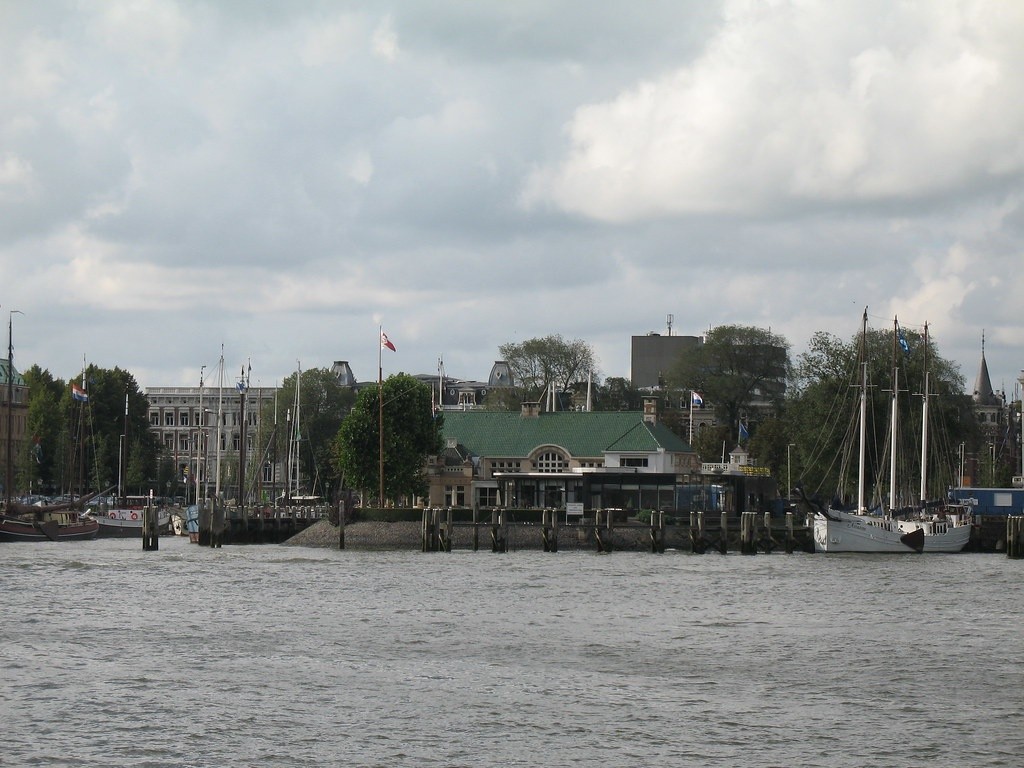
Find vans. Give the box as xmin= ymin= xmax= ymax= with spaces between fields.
xmin=1011 ymin=476 xmax=1022 ymax=488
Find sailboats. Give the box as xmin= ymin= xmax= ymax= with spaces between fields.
xmin=0 ymin=310 xmax=332 ymax=543
xmin=791 ymin=306 xmax=972 ymax=552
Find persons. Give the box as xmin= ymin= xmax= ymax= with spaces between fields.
xmin=512 ymin=495 xmax=516 ymax=507
xmin=265 ymin=505 xmax=271 ymax=519
xmin=947 ymin=483 xmax=955 ymax=504
xmin=47 ymin=513 xmax=53 ymax=522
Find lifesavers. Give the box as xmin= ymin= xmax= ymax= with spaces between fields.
xmin=131 ymin=513 xmax=138 ymax=519
xmin=109 ymin=513 xmax=116 ymax=519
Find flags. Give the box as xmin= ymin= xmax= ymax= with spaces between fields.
xmin=32 ymin=433 xmax=40 ymax=444
xmin=739 ymin=422 xmax=749 ymax=439
xmin=72 ymin=382 xmax=88 ymax=401
xmin=30 ymin=442 xmax=43 ymax=464
xmin=381 ymin=331 xmax=396 ymax=353
xmin=898 ymin=328 xmax=909 ymax=352
xmin=692 ymin=390 xmax=705 ymax=407
xmin=183 ymin=466 xmax=189 ymax=484
